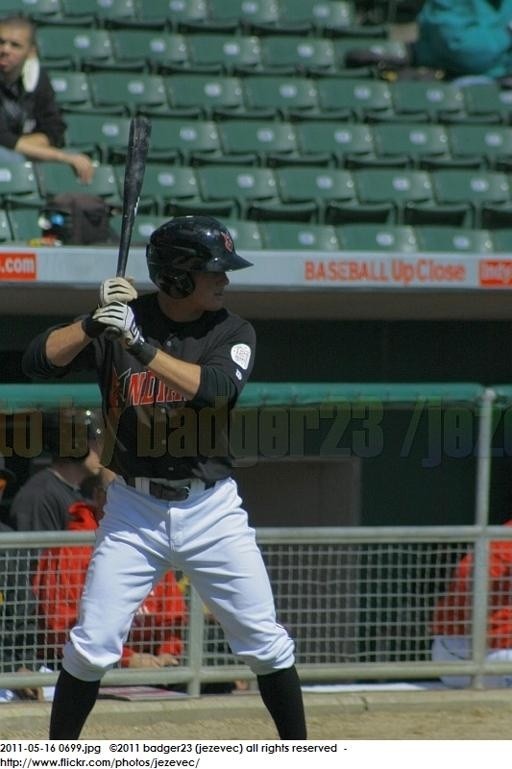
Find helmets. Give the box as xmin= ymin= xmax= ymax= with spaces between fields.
xmin=46 ymin=409 xmax=110 ymax=465
xmin=147 ymin=215 xmax=254 ymax=275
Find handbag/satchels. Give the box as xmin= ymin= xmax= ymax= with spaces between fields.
xmin=43 ymin=191 xmax=115 ymax=248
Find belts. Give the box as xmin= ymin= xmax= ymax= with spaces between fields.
xmin=121 ymin=472 xmax=217 ymax=502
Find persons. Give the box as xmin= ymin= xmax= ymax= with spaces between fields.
xmin=0 ymin=15 xmax=95 ymax=187
xmin=7 ymin=409 xmax=108 ymax=531
xmin=31 ymin=476 xmax=243 ymax=695
xmin=429 ymin=520 xmax=512 ymax=689
xmin=0 ymin=456 xmax=59 ymax=700
xmin=21 ymin=213 xmax=306 ymax=740
xmin=410 ymin=0 xmax=511 ymax=94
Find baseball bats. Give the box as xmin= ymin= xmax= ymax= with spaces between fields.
xmin=106 ymin=117 xmax=151 ymax=341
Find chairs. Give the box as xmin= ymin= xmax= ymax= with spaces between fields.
xmin=1 ymin=3 xmax=510 ymax=254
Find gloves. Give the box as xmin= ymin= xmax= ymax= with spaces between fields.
xmin=95 ymin=276 xmax=139 ymax=309
xmin=92 ymin=301 xmax=146 ymax=355
